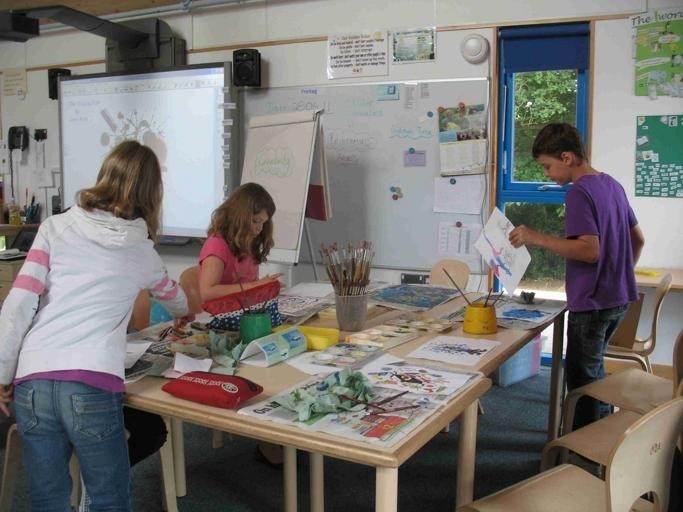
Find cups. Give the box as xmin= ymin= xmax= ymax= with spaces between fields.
xmin=521 ymin=292 xmax=535 ymax=304
xmin=334 ymin=296 xmax=367 ymax=330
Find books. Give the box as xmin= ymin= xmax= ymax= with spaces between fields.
xmin=381 ymin=312 xmax=459 ymax=336
xmin=343 ymin=323 xmax=419 ymax=355
xmin=122 ymin=358 xmax=153 ymax=378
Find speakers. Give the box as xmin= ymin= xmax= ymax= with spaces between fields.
xmin=47 ymin=68 xmax=71 ymax=100
xmin=232 ymin=48 xmax=261 ymax=88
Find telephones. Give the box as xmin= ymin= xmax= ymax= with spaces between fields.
xmin=7 ymin=126 xmax=28 ymax=150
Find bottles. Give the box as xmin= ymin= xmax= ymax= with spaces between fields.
xmin=463 ymin=303 xmax=498 ymax=334
xmin=240 ymin=310 xmax=271 ymax=344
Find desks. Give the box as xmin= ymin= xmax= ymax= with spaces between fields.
xmin=634 ymin=276 xmax=679 ymax=289
xmin=110 ymin=282 xmax=574 ymax=511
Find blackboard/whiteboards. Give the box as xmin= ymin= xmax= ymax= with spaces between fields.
xmin=237 ymin=77 xmax=498 ymax=273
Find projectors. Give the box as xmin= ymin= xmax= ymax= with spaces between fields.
xmin=0 ymin=13 xmax=40 ymax=42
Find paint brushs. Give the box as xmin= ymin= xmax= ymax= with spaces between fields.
xmin=319 ymin=241 xmax=375 ymax=330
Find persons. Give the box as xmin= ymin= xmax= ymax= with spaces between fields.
xmin=196 ymin=181 xmax=286 ymax=306
xmin=507 ymin=120 xmax=646 ymax=479
xmin=67 ymin=287 xmax=169 ymax=512
xmin=0 ymin=139 xmax=192 ymax=512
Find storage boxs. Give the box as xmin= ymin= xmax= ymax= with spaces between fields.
xmin=492 ymin=333 xmax=541 ymax=387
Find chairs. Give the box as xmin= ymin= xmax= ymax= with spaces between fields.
xmin=560 ymin=333 xmax=682 ymax=465
xmin=459 ymin=398 xmax=680 ymax=511
xmin=540 ymin=386 xmax=682 ymax=499
xmin=179 ymin=265 xmax=242 ymax=448
xmin=561 ymin=276 xmax=673 ymax=402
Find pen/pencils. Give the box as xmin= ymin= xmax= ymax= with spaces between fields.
xmin=338 ymin=391 xmax=422 ymax=415
xmin=234 ymin=276 xmax=267 ymax=313
xmin=158 ymin=326 xmax=193 ymax=342
xmin=23 ymin=196 xmax=39 ymax=217
xmin=441 ymin=267 xmax=504 ymax=308
